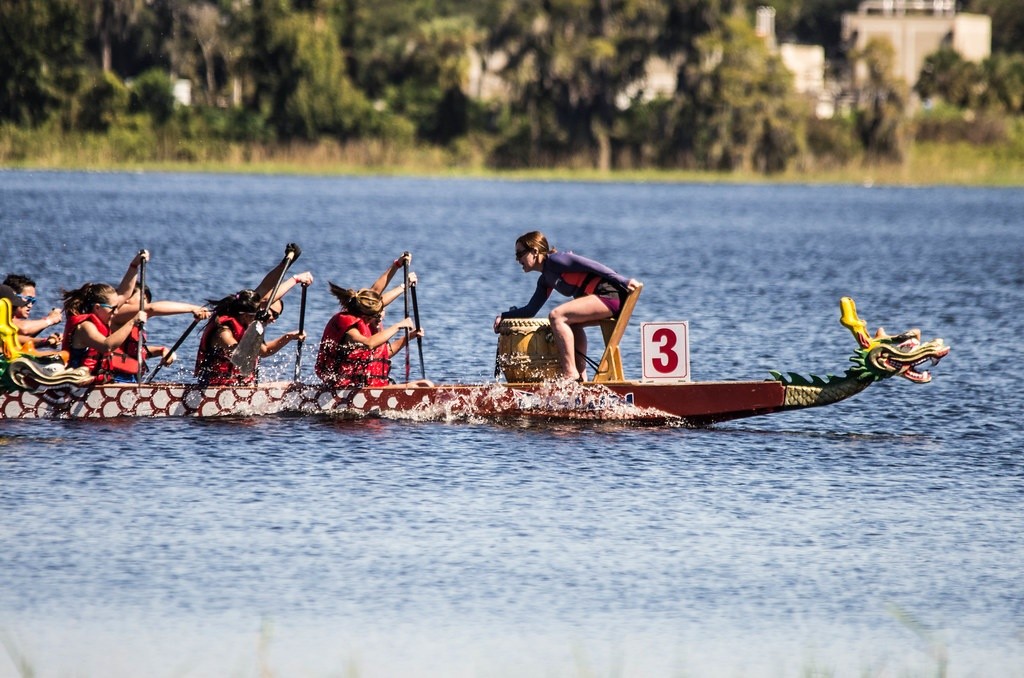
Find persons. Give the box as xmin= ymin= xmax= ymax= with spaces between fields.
xmin=314 ymin=252 xmax=425 ymax=387
xmin=193 ymin=243 xmax=314 ymax=387
xmin=0 ymin=273 xmax=69 ymax=371
xmin=62 ymin=249 xmax=211 ymax=383
xmin=494 ymin=229 xmax=635 ymax=381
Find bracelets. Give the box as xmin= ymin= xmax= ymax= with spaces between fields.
xmin=42 ymin=337 xmax=47 ymax=346
xmin=402 ymin=283 xmax=405 ymax=289
xmin=43 ymin=315 xmax=52 ymax=325
xmin=394 ymin=258 xmax=403 ymax=268
xmin=291 ymin=273 xmax=301 ymax=283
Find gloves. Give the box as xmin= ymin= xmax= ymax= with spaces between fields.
xmin=401 ymin=272 xmax=417 ymax=290
xmin=284 ymin=242 xmax=301 ymax=265
xmin=255 ymin=310 xmax=271 ymax=322
xmin=394 ymin=251 xmax=411 ymax=268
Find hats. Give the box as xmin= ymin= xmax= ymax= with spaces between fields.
xmin=0 ymin=284 xmax=29 ymax=307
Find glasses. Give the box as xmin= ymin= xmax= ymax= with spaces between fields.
xmin=367 ymin=305 xmax=383 ymax=319
xmin=25 ymin=296 xmax=36 ymax=305
xmin=270 ymin=308 xmax=279 ymax=319
xmin=92 ymin=302 xmax=118 ymax=310
xmin=516 ymin=248 xmax=529 ymax=258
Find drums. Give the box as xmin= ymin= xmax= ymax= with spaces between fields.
xmin=495 ymin=315 xmax=590 ymax=382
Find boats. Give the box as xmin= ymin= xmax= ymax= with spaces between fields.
xmin=0 ymin=296 xmax=951 ymax=426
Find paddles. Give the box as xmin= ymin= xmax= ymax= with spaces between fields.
xmin=230 ymin=246 xmax=295 ymax=373
xmin=127 ymin=258 xmax=152 ymax=389
xmin=288 ymin=283 xmax=311 ymax=387
xmin=142 ymin=317 xmax=203 ymax=382
xmin=400 ymin=251 xmax=411 ymax=388
xmin=410 ymin=281 xmax=432 ymax=382
